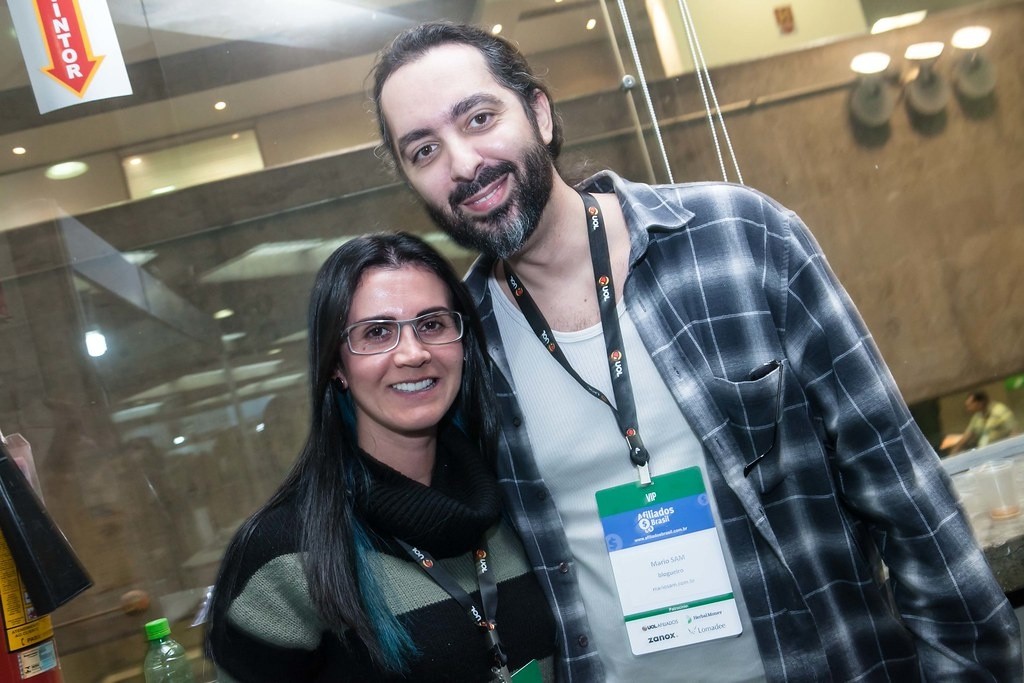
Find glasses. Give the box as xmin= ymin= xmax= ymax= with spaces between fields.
xmin=342 ymin=308 xmax=465 ymax=356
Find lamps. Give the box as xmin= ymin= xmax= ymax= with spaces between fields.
xmin=850 ymin=24 xmax=996 ymax=128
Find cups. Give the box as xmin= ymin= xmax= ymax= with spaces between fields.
xmin=969 ymin=457 xmax=1020 ymax=517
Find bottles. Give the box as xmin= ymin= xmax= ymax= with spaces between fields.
xmin=142 ymin=618 xmax=194 ymax=683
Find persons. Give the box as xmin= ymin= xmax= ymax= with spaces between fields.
xmin=202 ymin=232 xmax=554 ymax=683
xmin=371 ymin=26 xmax=1024 ymax=683
xmin=950 ymin=390 xmax=1020 ymax=451
